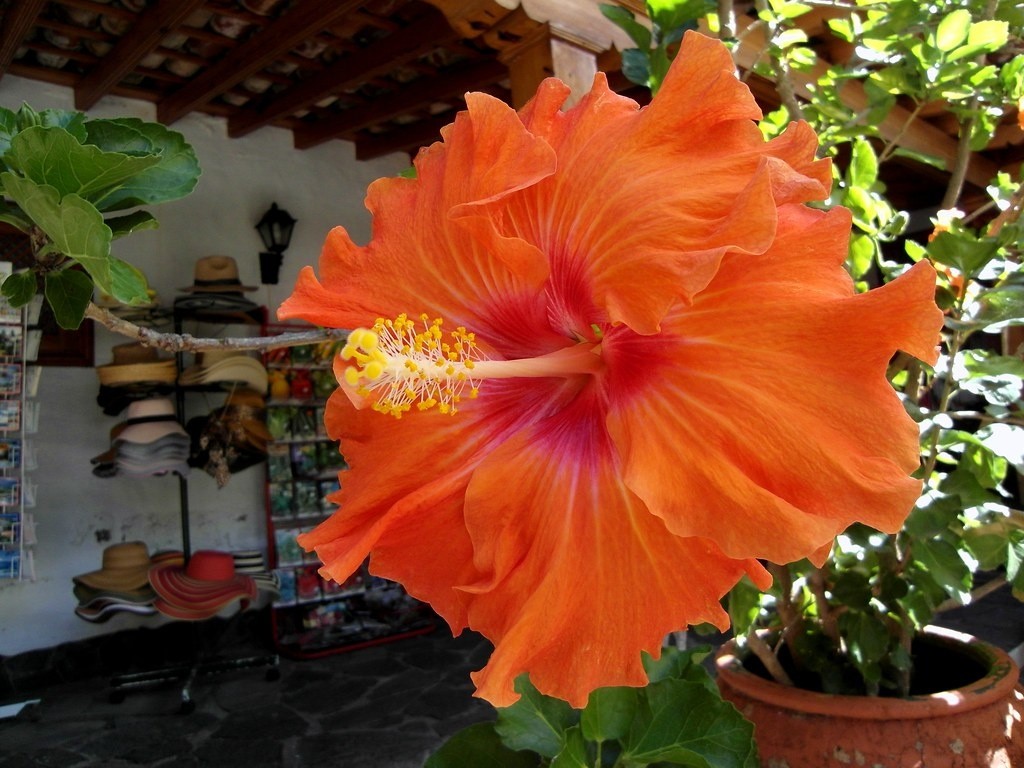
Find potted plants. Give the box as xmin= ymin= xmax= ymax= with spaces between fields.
xmin=599 ymin=1 xmax=1023 ymax=767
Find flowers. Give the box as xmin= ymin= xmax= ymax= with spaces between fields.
xmin=272 ymin=30 xmax=945 ymax=710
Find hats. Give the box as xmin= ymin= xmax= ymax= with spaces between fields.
xmin=90 ymin=396 xmax=192 ymax=481
xmin=94 ymin=342 xmax=178 ymax=416
xmin=73 ymin=542 xmax=281 ymax=621
xmin=179 ymin=254 xmax=259 ymax=310
xmin=179 ymin=350 xmax=269 ymax=395
xmin=188 ymin=399 xmax=275 ymax=456
xmin=100 ymin=286 xmax=167 ymax=328
